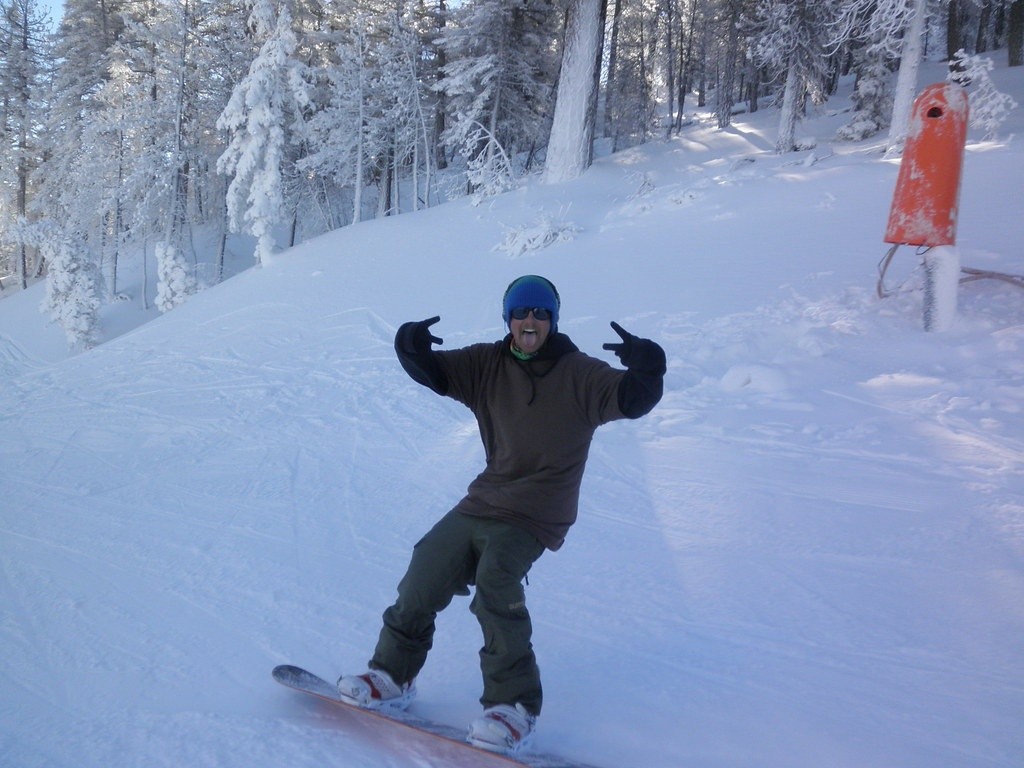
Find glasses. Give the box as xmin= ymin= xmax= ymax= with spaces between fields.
xmin=510 ymin=306 xmax=551 ymax=320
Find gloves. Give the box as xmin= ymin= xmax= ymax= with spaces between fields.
xmin=402 ymin=315 xmax=443 ymax=355
xmin=602 ymin=321 xmax=666 ymax=375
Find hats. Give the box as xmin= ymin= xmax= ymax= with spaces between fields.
xmin=502 ymin=275 xmax=561 ymax=337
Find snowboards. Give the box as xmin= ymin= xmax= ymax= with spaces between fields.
xmin=271 ymin=664 xmax=601 ymax=768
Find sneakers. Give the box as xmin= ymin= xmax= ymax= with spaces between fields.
xmin=466 ymin=701 xmax=537 ymax=753
xmin=337 ymin=667 xmax=417 ymax=710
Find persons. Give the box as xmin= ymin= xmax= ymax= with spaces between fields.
xmin=340 ymin=274 xmax=667 ymax=745
